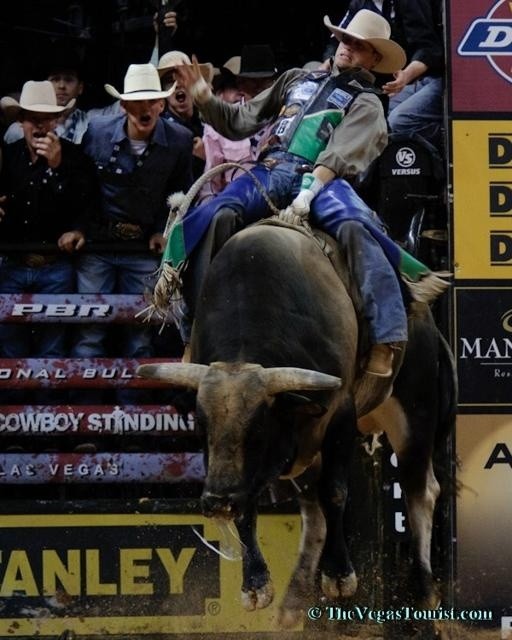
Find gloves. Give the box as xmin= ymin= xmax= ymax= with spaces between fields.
xmin=292 ymin=173 xmax=324 ymax=216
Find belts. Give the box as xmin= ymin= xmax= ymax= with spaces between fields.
xmin=116 ymin=222 xmax=141 ymax=240
xmin=30 ymin=254 xmax=45 ymax=265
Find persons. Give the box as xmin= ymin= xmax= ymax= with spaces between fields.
xmin=2 ymin=0 xmax=453 ymax=379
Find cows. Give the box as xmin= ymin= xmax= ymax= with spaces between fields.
xmin=136 ymin=210 xmax=458 ymax=630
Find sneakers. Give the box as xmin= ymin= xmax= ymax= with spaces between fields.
xmin=365 ymin=343 xmax=394 ymax=374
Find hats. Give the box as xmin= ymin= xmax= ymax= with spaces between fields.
xmin=0 ymin=80 xmax=76 ymax=113
xmin=323 ymin=8 xmax=407 ymax=74
xmin=104 ymin=51 xmax=242 ymax=101
